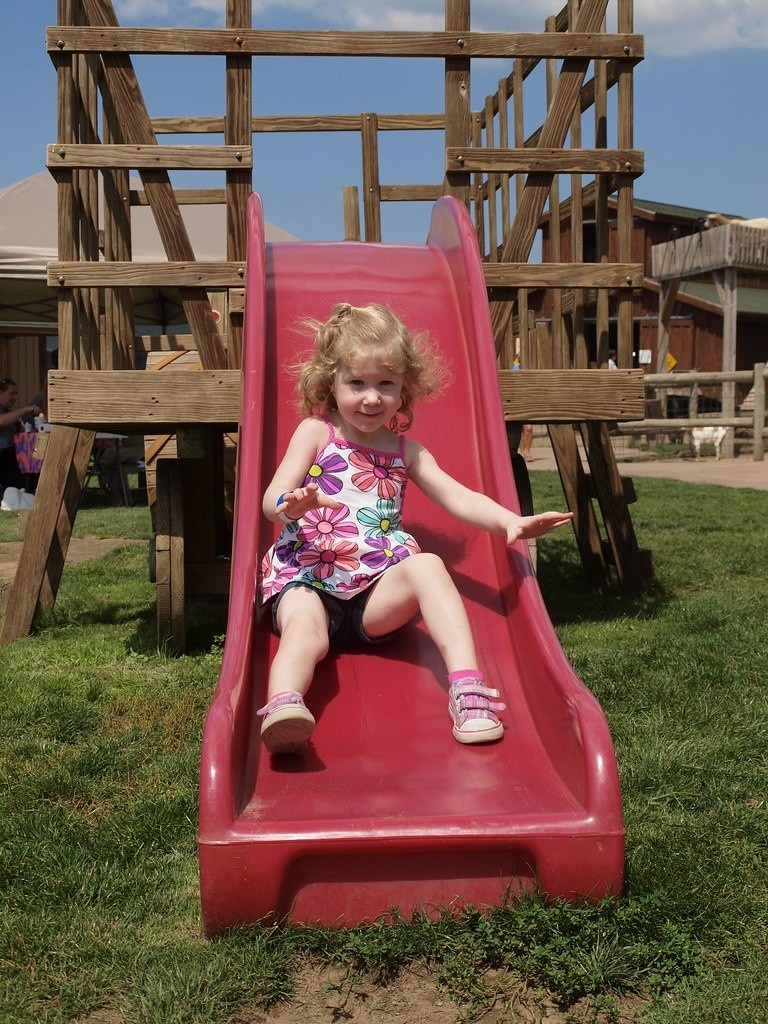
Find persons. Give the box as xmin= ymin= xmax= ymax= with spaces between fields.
xmin=257 ymin=303 xmax=574 ymax=756
xmin=0 ymin=371 xmax=44 ymax=503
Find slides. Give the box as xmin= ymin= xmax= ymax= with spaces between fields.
xmin=196 ymin=189 xmax=629 ymax=940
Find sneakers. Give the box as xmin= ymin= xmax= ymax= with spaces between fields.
xmin=256 ymin=690 xmax=312 ymax=755
xmin=447 ymin=680 xmax=504 ymax=742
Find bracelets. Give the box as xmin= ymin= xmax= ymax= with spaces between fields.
xmin=276 ymin=492 xmax=296 ymax=523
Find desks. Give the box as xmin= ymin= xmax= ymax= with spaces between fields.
xmin=36 ymin=422 xmax=129 ymax=507
